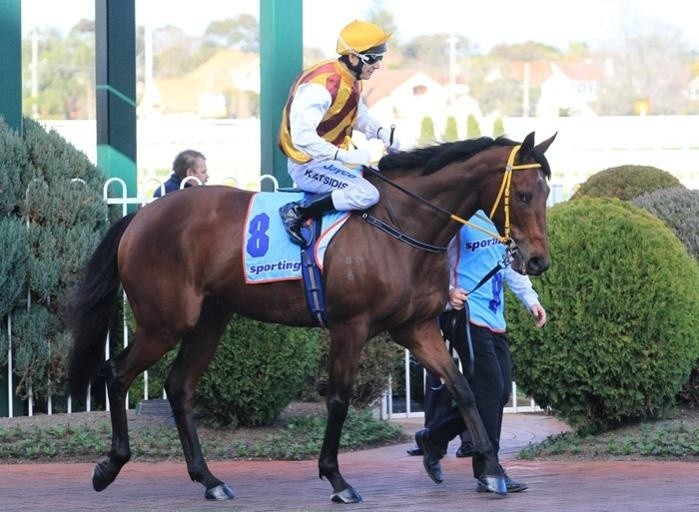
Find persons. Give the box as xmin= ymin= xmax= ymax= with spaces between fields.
xmin=407 ymin=368 xmax=474 ymax=458
xmin=414 ymin=206 xmax=547 ymax=495
xmin=276 ymin=16 xmax=402 ymax=245
xmin=152 ymin=150 xmax=210 ymax=198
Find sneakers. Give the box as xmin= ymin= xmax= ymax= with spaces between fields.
xmin=278 ymin=198 xmax=308 ymax=249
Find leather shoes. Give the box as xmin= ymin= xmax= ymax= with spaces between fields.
xmin=476 ymin=469 xmax=529 ymax=494
xmin=414 ymin=427 xmax=448 ymax=483
xmin=406 ymin=442 xmax=473 ymax=457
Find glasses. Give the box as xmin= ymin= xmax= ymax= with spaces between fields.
xmin=352 ymin=52 xmax=384 ymax=67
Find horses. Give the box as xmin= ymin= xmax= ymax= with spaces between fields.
xmin=63 ymin=129 xmax=558 ymax=504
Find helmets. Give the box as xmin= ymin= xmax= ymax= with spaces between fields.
xmin=335 ymin=18 xmax=397 ymax=53
